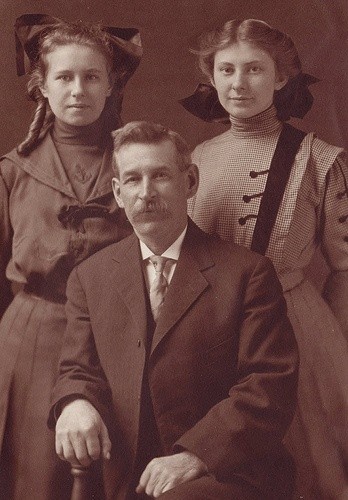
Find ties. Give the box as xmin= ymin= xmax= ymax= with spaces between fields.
xmin=150 ymin=255 xmax=168 ymax=324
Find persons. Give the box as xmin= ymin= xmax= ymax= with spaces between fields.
xmin=0 ymin=13 xmax=144 ymax=500
xmin=47 ymin=121 xmax=299 ymax=500
xmin=178 ymin=18 xmax=348 ymax=500
